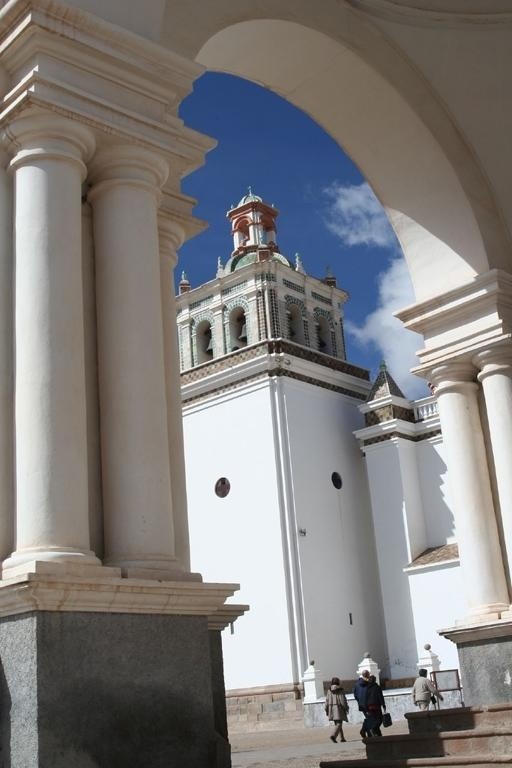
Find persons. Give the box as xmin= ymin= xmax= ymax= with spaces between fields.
xmin=412 ymin=669 xmax=443 ymax=711
xmin=326 ymin=677 xmax=349 ymax=743
xmin=354 ymin=670 xmax=371 ymax=738
xmin=365 ymin=675 xmax=386 ymax=735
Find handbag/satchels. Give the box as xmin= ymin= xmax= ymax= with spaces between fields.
xmin=431 ymin=695 xmax=436 ymax=704
xmin=382 ymin=713 xmax=392 ymax=727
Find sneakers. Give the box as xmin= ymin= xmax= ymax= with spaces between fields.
xmin=330 ymin=736 xmax=346 ymax=742
xmin=360 ymin=731 xmax=366 ymax=737
